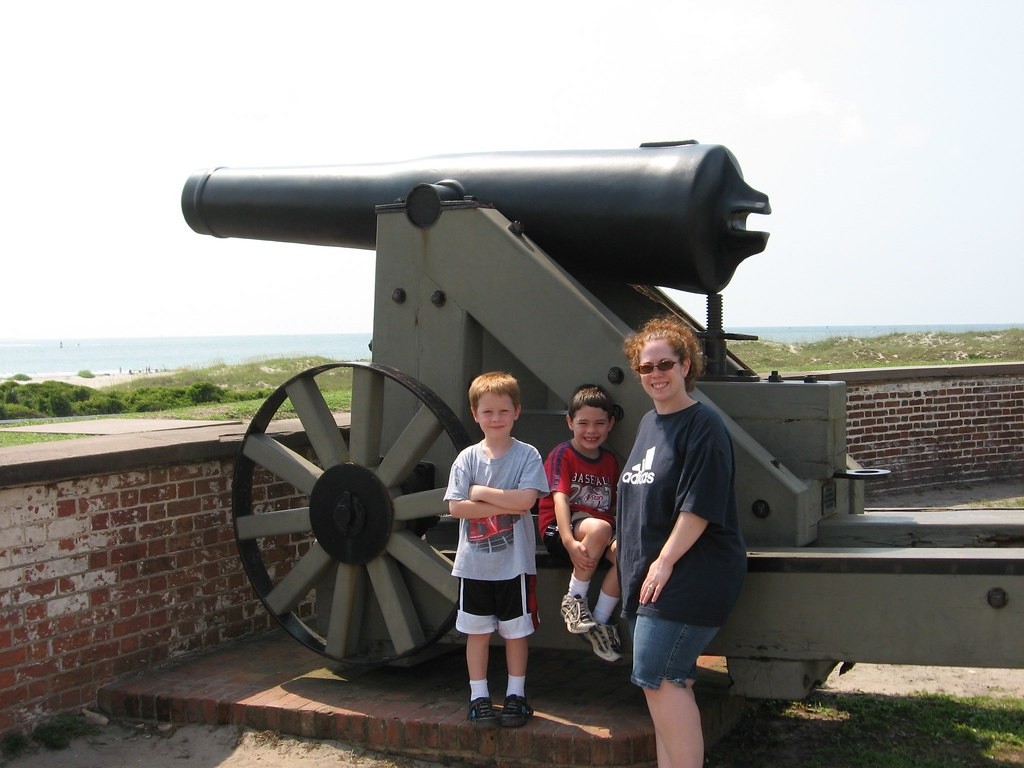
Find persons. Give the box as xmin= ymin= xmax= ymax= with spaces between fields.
xmin=536 ymin=383 xmax=624 ymax=665
xmin=442 ymin=372 xmax=550 ymax=731
xmin=613 ymin=315 xmax=747 ymax=768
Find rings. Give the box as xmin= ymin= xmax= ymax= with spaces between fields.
xmin=650 ymin=586 xmax=655 ymax=589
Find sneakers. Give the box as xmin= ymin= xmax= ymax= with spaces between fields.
xmin=560 ymin=595 xmax=599 ymax=633
xmin=500 ymin=690 xmax=533 ymax=727
xmin=578 ymin=621 xmax=624 ymax=664
xmin=468 ymin=696 xmax=497 ymax=728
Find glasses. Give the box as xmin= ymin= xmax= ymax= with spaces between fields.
xmin=635 ymin=360 xmax=680 ymax=374
